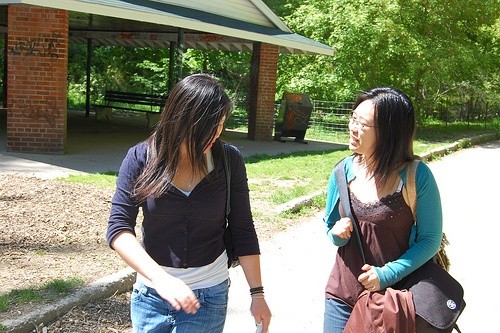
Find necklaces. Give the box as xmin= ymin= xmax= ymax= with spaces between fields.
xmin=174 ymin=166 xmax=195 ymax=191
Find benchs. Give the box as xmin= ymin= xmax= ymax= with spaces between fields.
xmin=91 ymin=90 xmax=170 ymax=130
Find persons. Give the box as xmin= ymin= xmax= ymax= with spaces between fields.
xmin=323 ymin=86 xmax=442 ymax=333
xmin=105 ymin=74 xmax=272 ymax=333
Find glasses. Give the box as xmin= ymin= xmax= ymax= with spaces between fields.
xmin=349 ymin=115 xmax=376 ymax=131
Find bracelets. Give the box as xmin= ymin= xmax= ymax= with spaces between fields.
xmin=249 ymin=286 xmax=264 ymax=299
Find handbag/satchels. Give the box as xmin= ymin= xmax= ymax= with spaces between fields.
xmin=221 ymin=143 xmax=241 ymax=267
xmin=404 ymin=262 xmax=466 ymax=333
